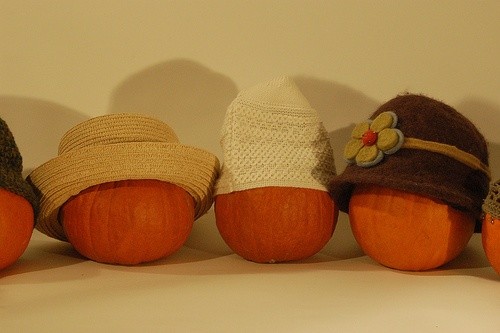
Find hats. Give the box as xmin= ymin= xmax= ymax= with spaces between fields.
xmin=483 ymin=177 xmax=499 ymax=220
xmin=330 ymin=94 xmax=489 ymax=234
xmin=214 ymin=77 xmax=338 ymax=195
xmin=25 ymin=113 xmax=221 ymax=242
xmin=0 ymin=119 xmax=38 ymax=228
